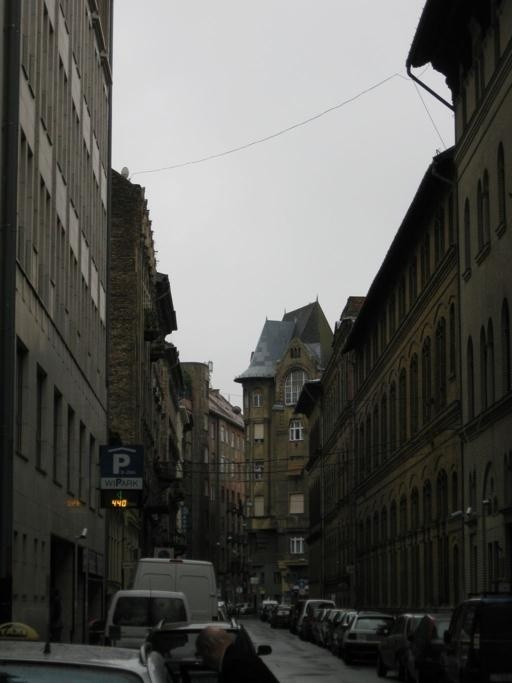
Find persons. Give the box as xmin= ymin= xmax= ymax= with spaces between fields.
xmin=196 ymin=627 xmax=280 ymax=683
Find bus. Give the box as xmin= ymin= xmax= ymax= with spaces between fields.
xmin=373 ymin=606 xmax=421 ymax=683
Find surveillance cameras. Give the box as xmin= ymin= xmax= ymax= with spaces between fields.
xmin=466 ymin=507 xmax=472 ymax=516
xmin=80 ymin=527 xmax=88 ymax=538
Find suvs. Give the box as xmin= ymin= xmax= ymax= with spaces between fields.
xmin=440 ymin=588 xmax=512 ymax=682
xmin=141 ymin=612 xmax=276 ymax=681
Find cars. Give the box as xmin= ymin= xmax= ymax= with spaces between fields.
xmin=0 ymin=616 xmax=177 ymax=681
xmin=410 ymin=606 xmax=452 ymax=680
xmin=218 ymin=588 xmax=391 ymax=664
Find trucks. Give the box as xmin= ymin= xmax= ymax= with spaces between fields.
xmin=134 ymin=554 xmax=222 ymax=624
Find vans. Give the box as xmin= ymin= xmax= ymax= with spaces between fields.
xmin=102 ymin=587 xmax=191 ymax=650
